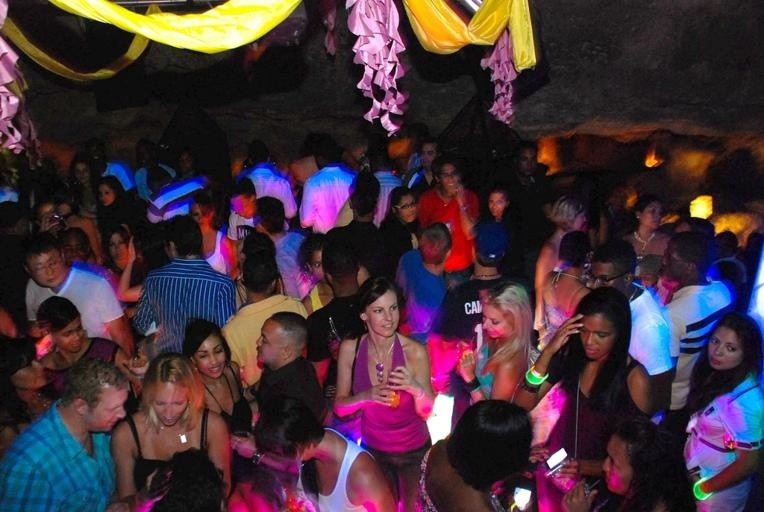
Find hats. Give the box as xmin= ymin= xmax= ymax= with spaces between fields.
xmin=469 ymin=220 xmax=508 ymax=259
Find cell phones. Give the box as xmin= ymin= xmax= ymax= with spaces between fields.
xmin=546 ymin=448 xmax=568 ymax=470
xmin=513 ymin=487 xmax=532 ymax=511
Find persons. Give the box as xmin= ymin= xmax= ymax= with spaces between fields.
xmin=1 ymin=138 xmax=764 ymax=512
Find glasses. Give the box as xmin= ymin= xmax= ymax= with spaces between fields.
xmin=392 ymin=200 xmax=418 ymax=211
xmin=439 ymin=171 xmax=460 ymax=180
xmin=587 ymin=270 xmax=630 ymax=287
